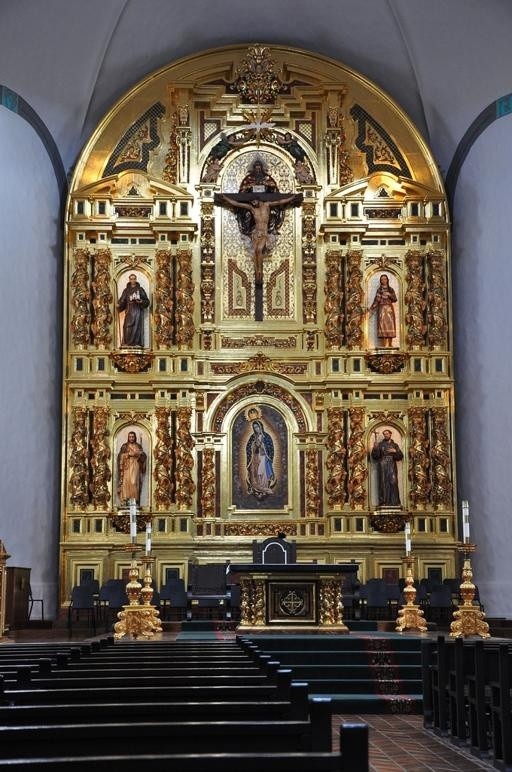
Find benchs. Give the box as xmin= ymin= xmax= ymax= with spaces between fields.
xmin=422 ymin=637 xmax=512 ymax=772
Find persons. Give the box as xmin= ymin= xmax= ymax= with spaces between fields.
xmin=239 ymin=161 xmax=276 ymax=193
xmin=115 ymin=273 xmax=149 ymax=347
xmin=199 ymin=134 xmax=243 ymax=183
xmin=371 ymin=430 xmax=404 ymax=506
xmin=219 ymin=192 xmax=298 ymax=285
xmin=245 ymin=421 xmax=277 ymax=502
xmin=117 ymin=432 xmax=146 ymax=506
xmin=368 ymin=274 xmax=398 ymax=347
xmin=276 ymin=131 xmax=317 ymax=185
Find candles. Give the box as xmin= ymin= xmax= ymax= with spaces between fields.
xmin=404 ymin=521 xmax=413 ymax=556
xmin=144 ymin=520 xmax=152 ymax=555
xmin=128 ymin=498 xmax=138 ymax=543
xmin=463 ymin=499 xmax=470 ymax=543
